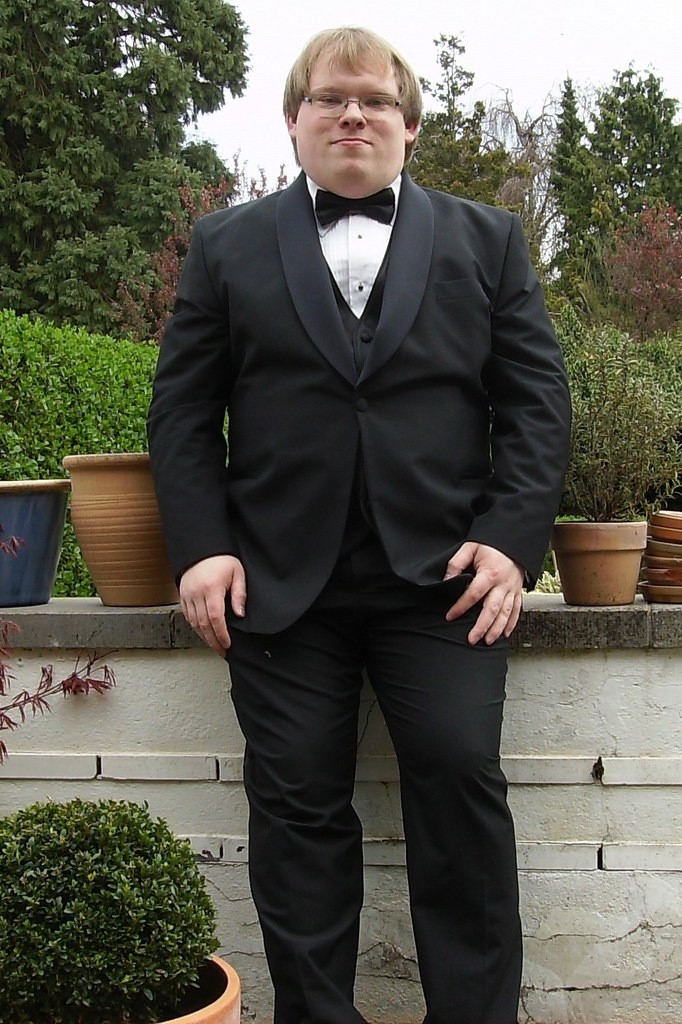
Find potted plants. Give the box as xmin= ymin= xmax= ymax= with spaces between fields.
xmin=0 ymin=797 xmax=242 ymax=1024
xmin=551 ymin=338 xmax=682 ymax=607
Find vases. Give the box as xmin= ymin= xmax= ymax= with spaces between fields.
xmin=0 ymin=478 xmax=71 ymax=608
xmin=61 ymin=452 xmax=182 ymax=607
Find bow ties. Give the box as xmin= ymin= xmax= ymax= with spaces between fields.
xmin=315 ymin=187 xmax=395 ymax=226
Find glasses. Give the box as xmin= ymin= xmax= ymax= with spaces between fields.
xmin=304 ymin=93 xmax=403 ymax=121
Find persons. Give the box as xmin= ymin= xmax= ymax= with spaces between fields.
xmin=147 ymin=26 xmax=573 ymax=1024
